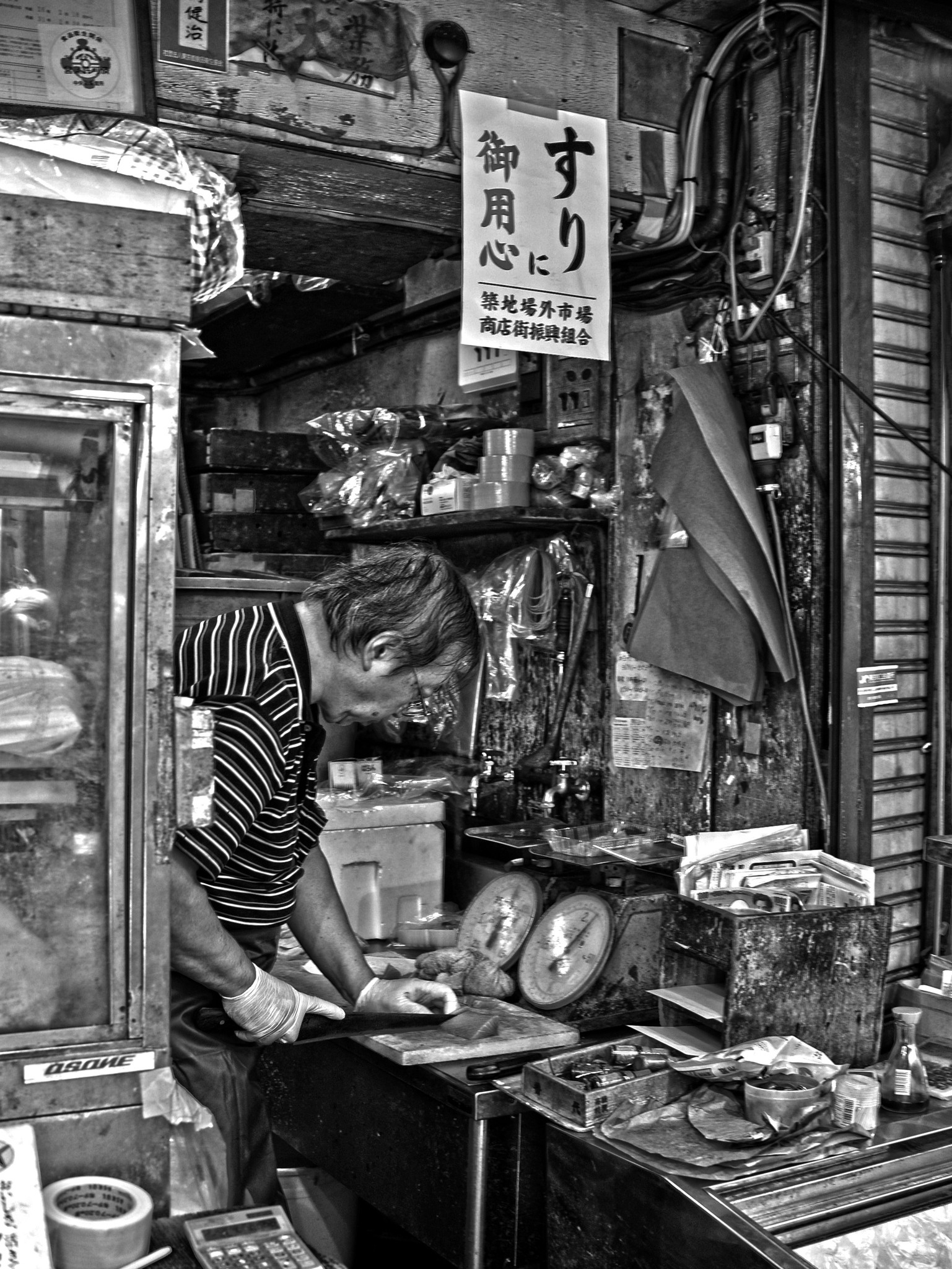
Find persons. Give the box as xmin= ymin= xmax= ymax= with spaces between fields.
xmin=72 ymin=542 xmax=492 ymax=1230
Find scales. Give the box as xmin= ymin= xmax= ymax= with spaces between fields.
xmin=511 ymin=821 xmax=697 ymax=1033
xmin=454 ymin=815 xmax=582 ymax=976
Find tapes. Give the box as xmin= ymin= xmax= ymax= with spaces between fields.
xmin=42 ymin=1175 xmax=154 ymax=1269
xmin=482 ymin=428 xmax=535 ymax=457
xmin=477 ymin=455 xmax=532 ymax=484
xmin=470 ymin=482 xmax=531 ymax=510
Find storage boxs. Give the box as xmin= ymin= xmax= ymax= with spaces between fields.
xmin=660 ymin=889 xmax=897 ymax=1068
xmin=277 ymin=791 xmax=445 ymax=945
xmin=186 ymin=424 xmax=347 ymax=570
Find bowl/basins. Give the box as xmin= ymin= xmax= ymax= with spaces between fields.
xmin=742 ymin=1074 xmax=822 ymax=1125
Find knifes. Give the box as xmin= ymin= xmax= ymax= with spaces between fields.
xmin=191 ymin=1002 xmax=453 ymax=1048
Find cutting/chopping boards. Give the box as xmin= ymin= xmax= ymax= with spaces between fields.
xmin=271 ymin=949 xmax=580 ymax=1067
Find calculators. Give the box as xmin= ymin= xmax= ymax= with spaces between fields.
xmin=183 ymin=1202 xmax=324 ymax=1269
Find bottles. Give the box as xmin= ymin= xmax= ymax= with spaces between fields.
xmin=880 ymin=1003 xmax=932 ymax=1118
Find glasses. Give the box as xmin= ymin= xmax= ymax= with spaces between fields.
xmin=396 ymin=640 xmax=432 ymax=723
